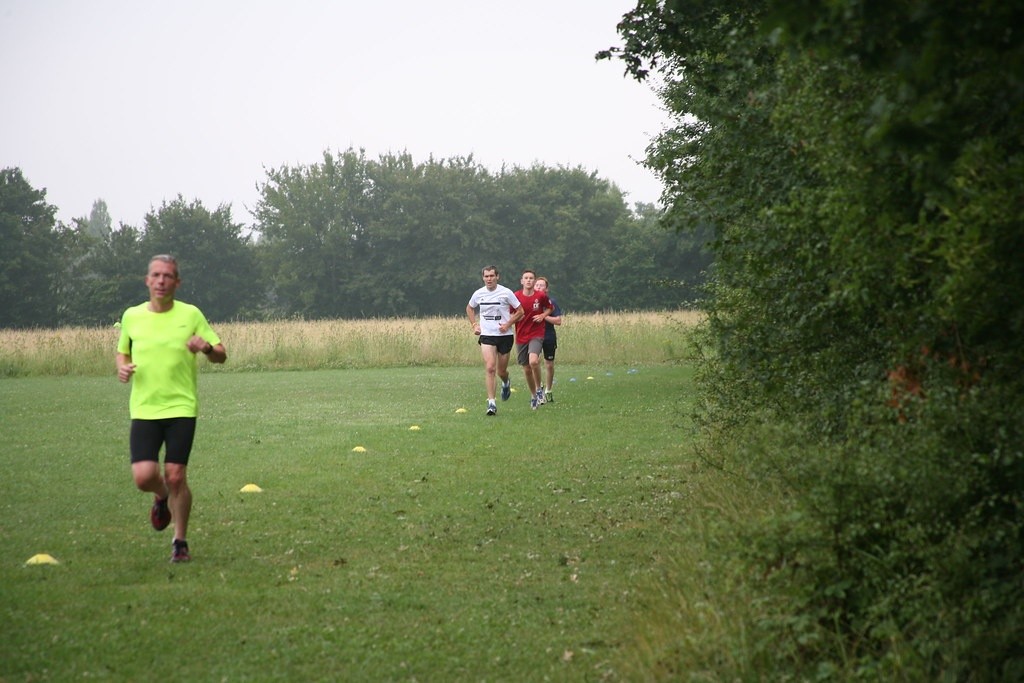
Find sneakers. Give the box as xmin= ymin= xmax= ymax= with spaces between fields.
xmin=500 ymin=379 xmax=510 ymax=400
xmin=151 ymin=478 xmax=172 ymax=531
xmin=487 ymin=403 xmax=497 ymax=415
xmin=531 ymin=394 xmax=539 ymax=411
xmin=172 ymin=538 xmax=190 ymax=563
xmin=535 ymin=385 xmax=547 ymax=405
xmin=545 ymin=389 xmax=553 ymax=402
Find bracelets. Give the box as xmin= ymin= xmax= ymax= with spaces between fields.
xmin=473 ymin=323 xmax=476 ymax=327
xmin=202 ymin=344 xmax=213 ymax=354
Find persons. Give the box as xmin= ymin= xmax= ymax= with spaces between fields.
xmin=534 ymin=277 xmax=563 ymax=402
xmin=115 ymin=254 xmax=227 ymax=563
xmin=510 ymin=270 xmax=554 ymax=410
xmin=466 ymin=266 xmax=524 ymax=416
